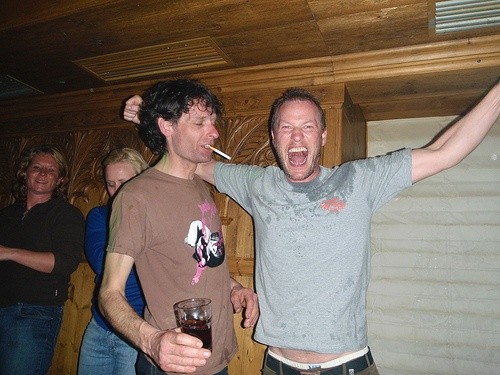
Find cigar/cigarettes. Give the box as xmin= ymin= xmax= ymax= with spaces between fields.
xmin=208 ymin=145 xmax=232 ymax=162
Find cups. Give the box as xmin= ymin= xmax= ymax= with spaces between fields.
xmin=174 ymin=298 xmax=212 ymax=352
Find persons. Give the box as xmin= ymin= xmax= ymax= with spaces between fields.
xmin=123 ymin=82 xmax=500 ymax=375
xmin=98 ymin=77 xmax=260 ymax=375
xmin=0 ymin=145 xmax=87 ymax=375
xmin=76 ymin=146 xmax=150 ymax=375
